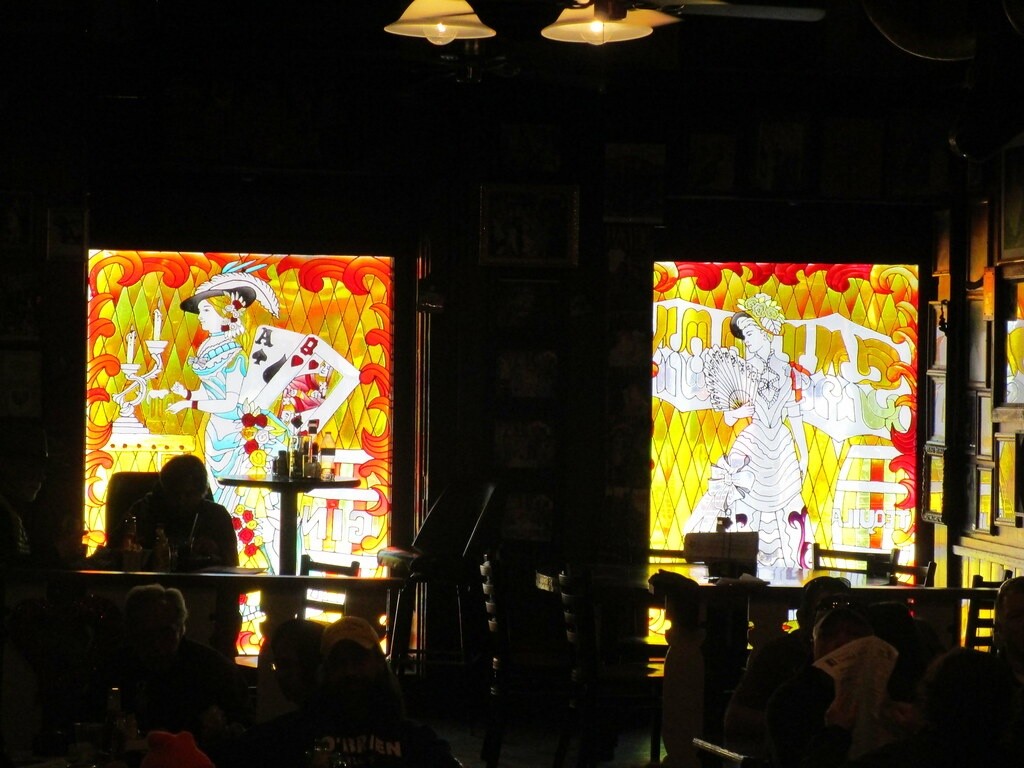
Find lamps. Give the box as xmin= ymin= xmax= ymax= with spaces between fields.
xmin=540 ymin=1 xmax=653 ymax=46
xmin=383 ymin=0 xmax=497 ymax=47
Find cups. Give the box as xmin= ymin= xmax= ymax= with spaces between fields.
xmin=297 ymin=430 xmax=308 ymax=452
xmin=302 ymin=454 xmax=316 ymax=478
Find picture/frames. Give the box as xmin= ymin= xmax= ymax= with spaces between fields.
xmin=471 ymin=177 xmax=582 ymax=272
xmin=920 ymin=133 xmax=1024 ymax=536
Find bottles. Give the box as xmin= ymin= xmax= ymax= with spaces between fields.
xmin=320 ymin=431 xmax=335 ymax=481
xmin=278 ymin=449 xmax=288 ymax=476
xmin=307 ymin=420 xmax=319 ymax=479
xmin=123 ymin=514 xmax=143 ymax=571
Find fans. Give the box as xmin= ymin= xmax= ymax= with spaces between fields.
xmin=633 ymin=0 xmax=828 ymax=23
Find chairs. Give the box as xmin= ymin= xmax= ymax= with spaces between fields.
xmin=475 ymin=550 xmax=1013 ymax=768
xmin=106 ymin=472 xmax=213 ymax=544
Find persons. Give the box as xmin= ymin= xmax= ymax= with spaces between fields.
xmin=110 ymin=457 xmax=240 ymax=656
xmin=724 ymin=576 xmax=1024 ymax=768
xmin=0 ymin=583 xmax=462 ymax=768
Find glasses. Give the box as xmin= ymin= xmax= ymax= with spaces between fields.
xmin=814 ymin=596 xmax=856 ymax=620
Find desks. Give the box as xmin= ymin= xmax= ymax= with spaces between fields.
xmin=217 ymin=474 xmax=362 ymax=567
xmin=597 ymin=565 xmax=888 ymax=766
xmin=35 ymin=571 xmax=402 ymax=666
